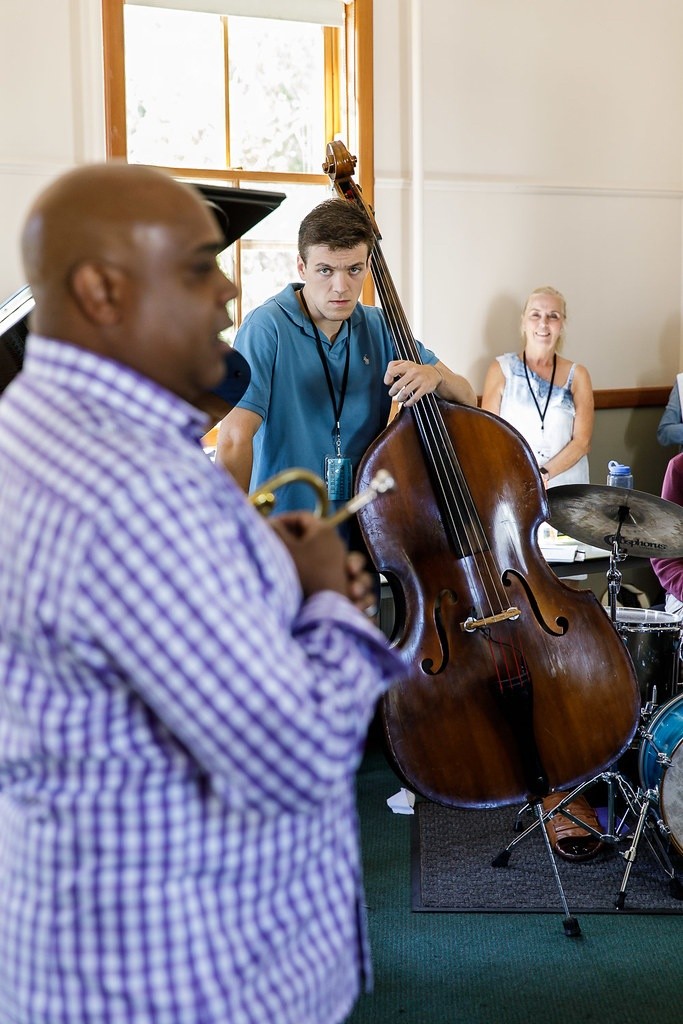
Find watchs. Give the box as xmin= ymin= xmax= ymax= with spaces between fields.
xmin=537 ymin=467 xmax=549 ymax=484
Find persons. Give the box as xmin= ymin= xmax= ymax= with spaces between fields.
xmin=489 ymin=286 xmax=594 ymax=491
xmin=0 ymin=165 xmax=415 ymax=1024
xmin=211 ymin=200 xmax=605 ymax=865
xmin=649 ymin=375 xmax=683 ymax=617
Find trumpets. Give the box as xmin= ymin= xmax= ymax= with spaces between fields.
xmin=247 ymin=467 xmax=400 ymax=528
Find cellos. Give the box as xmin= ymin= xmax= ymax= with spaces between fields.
xmin=321 ymin=139 xmax=643 ymax=815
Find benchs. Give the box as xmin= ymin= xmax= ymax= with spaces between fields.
xmin=475 ymin=385 xmax=675 ymax=603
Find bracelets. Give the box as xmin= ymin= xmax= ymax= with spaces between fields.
xmin=432 ymin=365 xmax=443 ymax=389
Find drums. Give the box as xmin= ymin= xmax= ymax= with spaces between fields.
xmin=638 ymin=691 xmax=683 ymax=875
xmin=602 ymin=606 xmax=682 ymax=706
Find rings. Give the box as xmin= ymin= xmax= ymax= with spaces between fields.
xmin=364 ymin=605 xmax=379 ymax=617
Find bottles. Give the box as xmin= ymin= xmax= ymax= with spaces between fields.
xmin=606 ymin=460 xmax=634 ymax=489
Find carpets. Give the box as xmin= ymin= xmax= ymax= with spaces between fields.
xmin=411 ymin=790 xmax=683 ymax=915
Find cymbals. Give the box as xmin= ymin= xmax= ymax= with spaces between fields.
xmin=545 ymin=483 xmax=683 ymax=558
